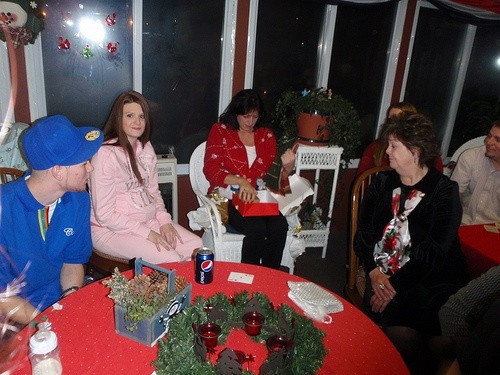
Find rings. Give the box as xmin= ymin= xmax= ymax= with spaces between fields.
xmin=379 ymin=285 xmax=384 ymax=289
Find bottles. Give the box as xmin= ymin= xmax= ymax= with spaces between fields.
xmin=28 ymin=323 xmax=63 ymax=375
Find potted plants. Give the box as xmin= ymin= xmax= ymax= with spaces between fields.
xmin=101 ymin=256 xmax=192 ymax=347
xmin=272 ymin=85 xmax=360 ymax=184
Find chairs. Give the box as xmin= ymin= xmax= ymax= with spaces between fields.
xmin=442 ymin=135 xmax=488 ymax=179
xmin=0 ymin=166 xmax=27 ymax=184
xmin=188 ymin=140 xmax=301 ymax=276
xmin=345 ymin=165 xmax=397 ymax=301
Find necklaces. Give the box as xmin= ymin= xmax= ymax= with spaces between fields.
xmin=400 ymin=180 xmax=407 ymax=192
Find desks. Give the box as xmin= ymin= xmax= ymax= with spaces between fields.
xmin=7 ymin=261 xmax=411 ymax=375
xmin=459 ymin=222 xmax=500 ymax=280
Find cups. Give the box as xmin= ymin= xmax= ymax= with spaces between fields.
xmin=242 ymin=311 xmax=265 ymax=336
xmin=198 ymin=322 xmax=222 ymax=348
xmin=267 ymin=336 xmax=292 ymax=358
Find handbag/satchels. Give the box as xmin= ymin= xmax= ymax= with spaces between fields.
xmin=287 ymin=280 xmax=344 ymax=324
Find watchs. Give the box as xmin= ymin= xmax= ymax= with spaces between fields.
xmin=59 ymin=286 xmax=79 ymax=298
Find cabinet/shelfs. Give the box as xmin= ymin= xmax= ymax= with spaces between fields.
xmin=154 ymin=154 xmax=179 ymax=224
xmin=292 ymin=142 xmax=344 ymax=258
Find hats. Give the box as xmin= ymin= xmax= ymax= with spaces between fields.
xmin=21 ymin=113 xmax=103 ymax=171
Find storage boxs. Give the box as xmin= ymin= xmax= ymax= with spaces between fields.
xmin=232 ymin=187 xmax=280 ymax=218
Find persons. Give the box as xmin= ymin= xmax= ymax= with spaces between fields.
xmin=87 ymin=90 xmax=203 ymax=264
xmin=356 ymin=102 xmax=444 ymax=205
xmin=203 ymin=89 xmax=295 ymax=270
xmin=354 ymin=109 xmax=475 ymax=375
xmin=0 ymin=115 xmax=104 ymax=324
xmin=449 ymin=122 xmax=500 ymax=226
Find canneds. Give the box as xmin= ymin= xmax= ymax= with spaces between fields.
xmin=195 ymin=247 xmax=214 ymax=284
